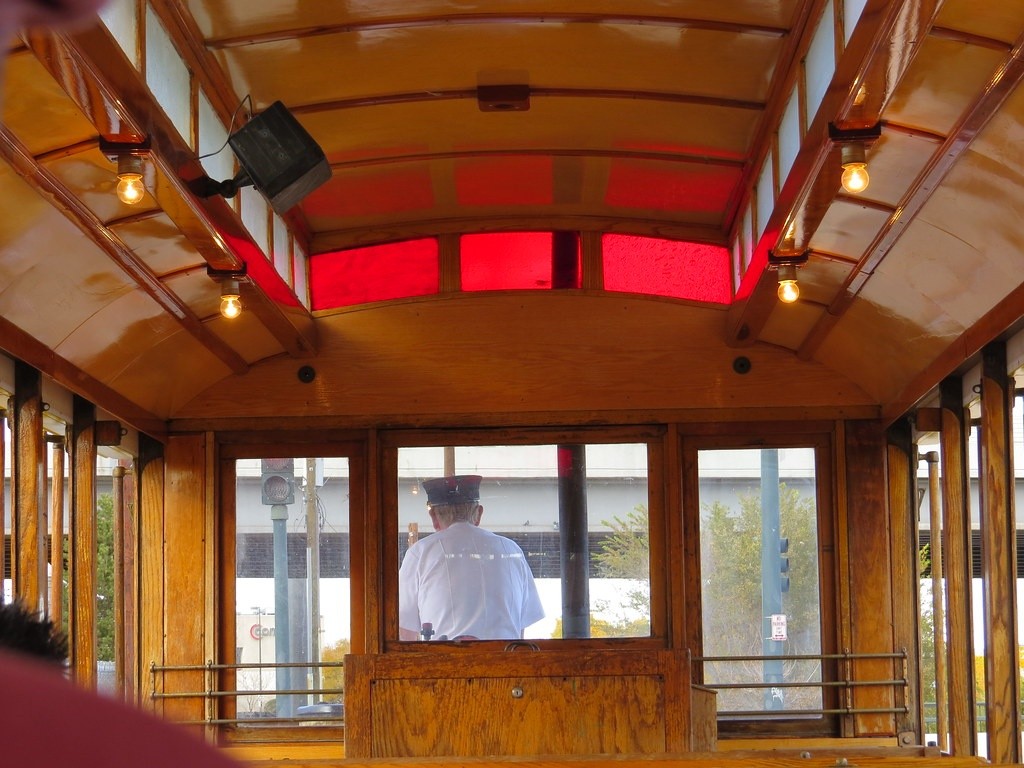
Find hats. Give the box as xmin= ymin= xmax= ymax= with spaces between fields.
xmin=422 ymin=475 xmax=482 ymax=506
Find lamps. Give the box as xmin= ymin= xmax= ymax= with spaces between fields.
xmin=825 ymin=121 xmax=881 ymax=199
xmin=760 ymin=248 xmax=818 ymax=309
xmin=201 ymin=262 xmax=253 ymax=325
xmin=92 ymin=131 xmax=157 ymax=206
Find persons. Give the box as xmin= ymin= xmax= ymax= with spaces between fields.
xmin=399 ymin=475 xmax=546 ymax=641
